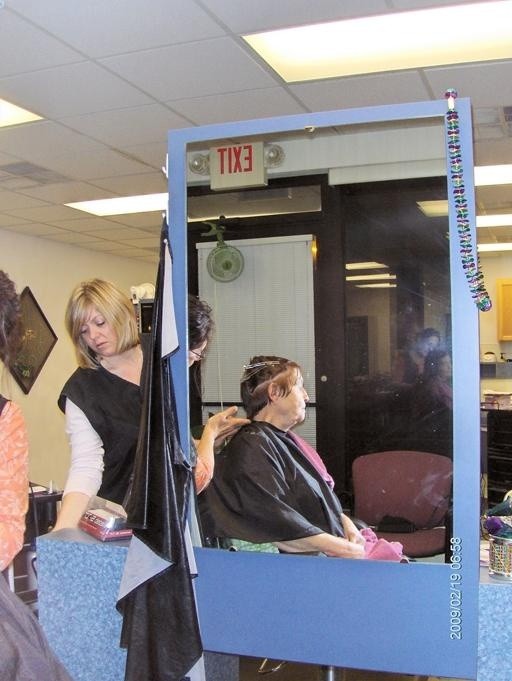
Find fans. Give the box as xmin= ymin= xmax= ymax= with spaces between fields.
xmin=199 ymin=220 xmax=245 ymax=283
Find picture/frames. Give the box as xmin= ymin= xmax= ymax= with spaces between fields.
xmin=0 ymin=286 xmax=58 ymax=395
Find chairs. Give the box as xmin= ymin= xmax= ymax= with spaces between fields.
xmin=352 ymin=450 xmax=453 ymax=558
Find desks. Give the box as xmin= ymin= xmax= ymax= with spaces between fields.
xmin=8 ymin=483 xmax=64 ymax=605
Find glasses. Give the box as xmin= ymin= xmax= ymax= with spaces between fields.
xmin=190 ymin=350 xmax=206 ymax=360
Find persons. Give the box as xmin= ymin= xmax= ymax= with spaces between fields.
xmin=403 ymin=328 xmax=441 ymax=383
xmin=0 ymin=269 xmax=29 ymax=574
xmin=220 ymin=356 xmax=367 ymax=560
xmin=410 ymin=350 xmax=452 ymax=411
xmin=188 ymin=294 xmax=250 ymax=497
xmin=50 ymin=279 xmax=153 ymax=531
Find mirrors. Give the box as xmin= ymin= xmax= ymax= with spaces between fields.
xmin=166 ymin=95 xmax=482 ymax=681
